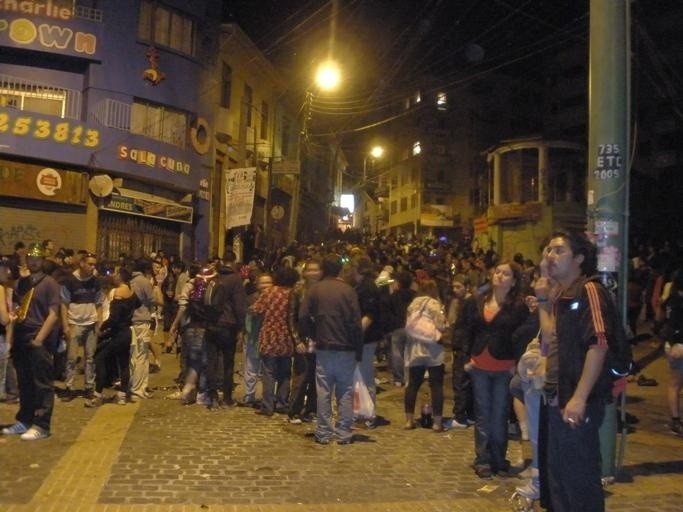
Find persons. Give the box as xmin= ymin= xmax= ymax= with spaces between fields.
xmin=160 ymin=233 xmax=379 ymax=427
xmin=297 ymin=256 xmax=366 ymax=444
xmin=3 ymin=241 xmax=60 ymax=440
xmin=628 ymin=226 xmax=681 ymax=429
xmin=533 ymin=227 xmax=618 ymax=511
xmin=366 ymin=227 xmax=556 ymax=498
xmin=0 ymin=240 xmax=165 ymax=408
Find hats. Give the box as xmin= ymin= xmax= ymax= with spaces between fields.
xmin=20 ymin=241 xmax=55 ymax=257
xmin=414 ymin=269 xmax=436 ymax=286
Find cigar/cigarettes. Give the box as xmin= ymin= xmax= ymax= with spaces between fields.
xmin=566 ymin=417 xmax=575 ymax=423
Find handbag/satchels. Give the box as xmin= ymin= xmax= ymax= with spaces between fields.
xmin=182 ymin=325 xmax=206 ymax=359
xmin=404 ymin=296 xmax=443 ymax=345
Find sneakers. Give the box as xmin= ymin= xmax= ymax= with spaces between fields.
xmin=402 ymin=415 xmax=475 ymax=433
xmin=669 ymin=415 xmax=683 ymax=435
xmin=2 ymin=420 xmax=49 ymax=441
xmin=60 ymin=389 xmax=152 ymax=408
xmin=166 ymin=388 xmax=312 ymax=425
xmin=474 ymin=462 xmax=540 ymax=500
xmin=507 ymin=419 xmax=520 ymax=435
xmin=311 ymin=416 xmax=381 ymax=445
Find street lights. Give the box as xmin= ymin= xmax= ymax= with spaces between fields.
xmin=287 ymin=59 xmax=341 ymax=248
xmin=362 ymin=145 xmax=383 ymax=181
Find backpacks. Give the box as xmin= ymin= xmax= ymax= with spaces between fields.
xmin=188 ymin=263 xmax=223 ymax=312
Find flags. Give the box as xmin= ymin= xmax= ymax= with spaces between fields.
xmin=222 ymin=165 xmax=258 ymax=231
